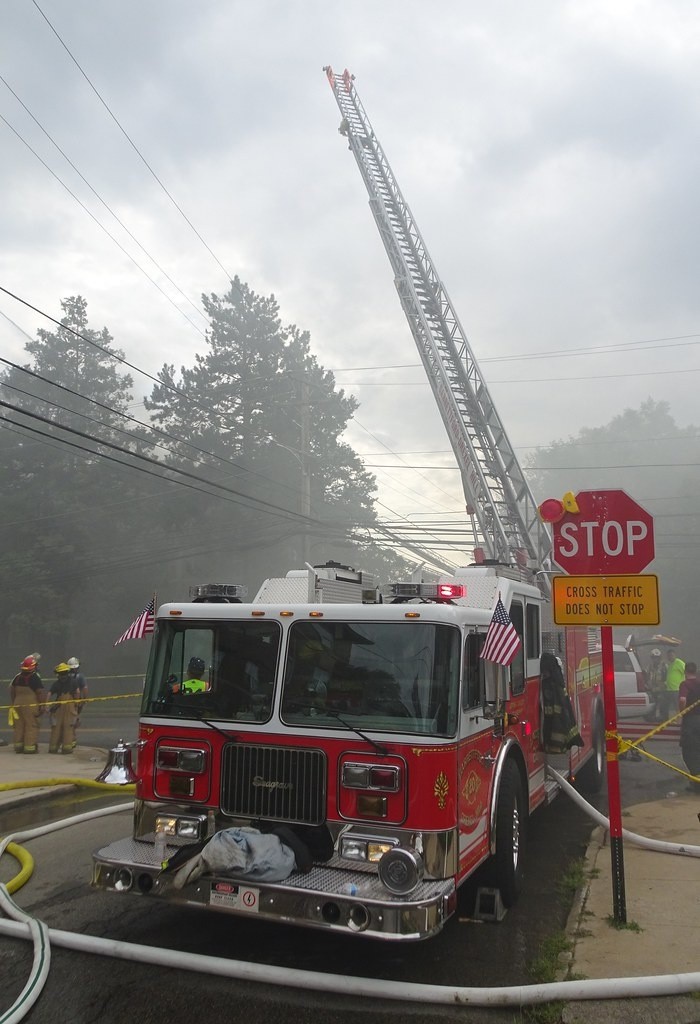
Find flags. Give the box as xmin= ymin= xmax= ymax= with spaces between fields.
xmin=113 ymin=598 xmax=155 ymax=647
xmin=480 ymin=600 xmax=521 ymax=668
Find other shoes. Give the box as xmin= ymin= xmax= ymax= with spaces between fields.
xmin=686 ymin=785 xmax=700 ymax=793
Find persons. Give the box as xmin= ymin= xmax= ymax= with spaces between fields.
xmin=679 ymin=662 xmax=700 ymax=795
xmin=46 ymin=657 xmax=88 ymax=754
xmin=171 ymin=656 xmax=209 ymax=693
xmin=664 ymin=650 xmax=686 ymax=725
xmin=646 ymin=649 xmax=673 ymax=723
xmin=9 ymin=652 xmax=45 ymax=755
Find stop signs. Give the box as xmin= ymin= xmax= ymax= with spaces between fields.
xmin=553 ymin=487 xmax=656 ymax=574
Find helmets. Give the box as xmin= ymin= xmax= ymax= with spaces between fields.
xmin=55 ymin=663 xmax=71 ymax=672
xmin=20 ymin=655 xmax=36 ymax=671
xmin=67 ymin=657 xmax=79 ymax=668
xmin=650 ymin=648 xmax=661 ymax=656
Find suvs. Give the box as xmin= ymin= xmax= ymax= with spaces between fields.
xmin=613 ymin=634 xmax=680 ymax=722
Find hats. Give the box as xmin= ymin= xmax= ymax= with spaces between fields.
xmin=188 ymin=657 xmax=205 ymax=675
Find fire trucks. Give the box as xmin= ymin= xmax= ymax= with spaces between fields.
xmin=90 ymin=64 xmax=602 ymax=943
